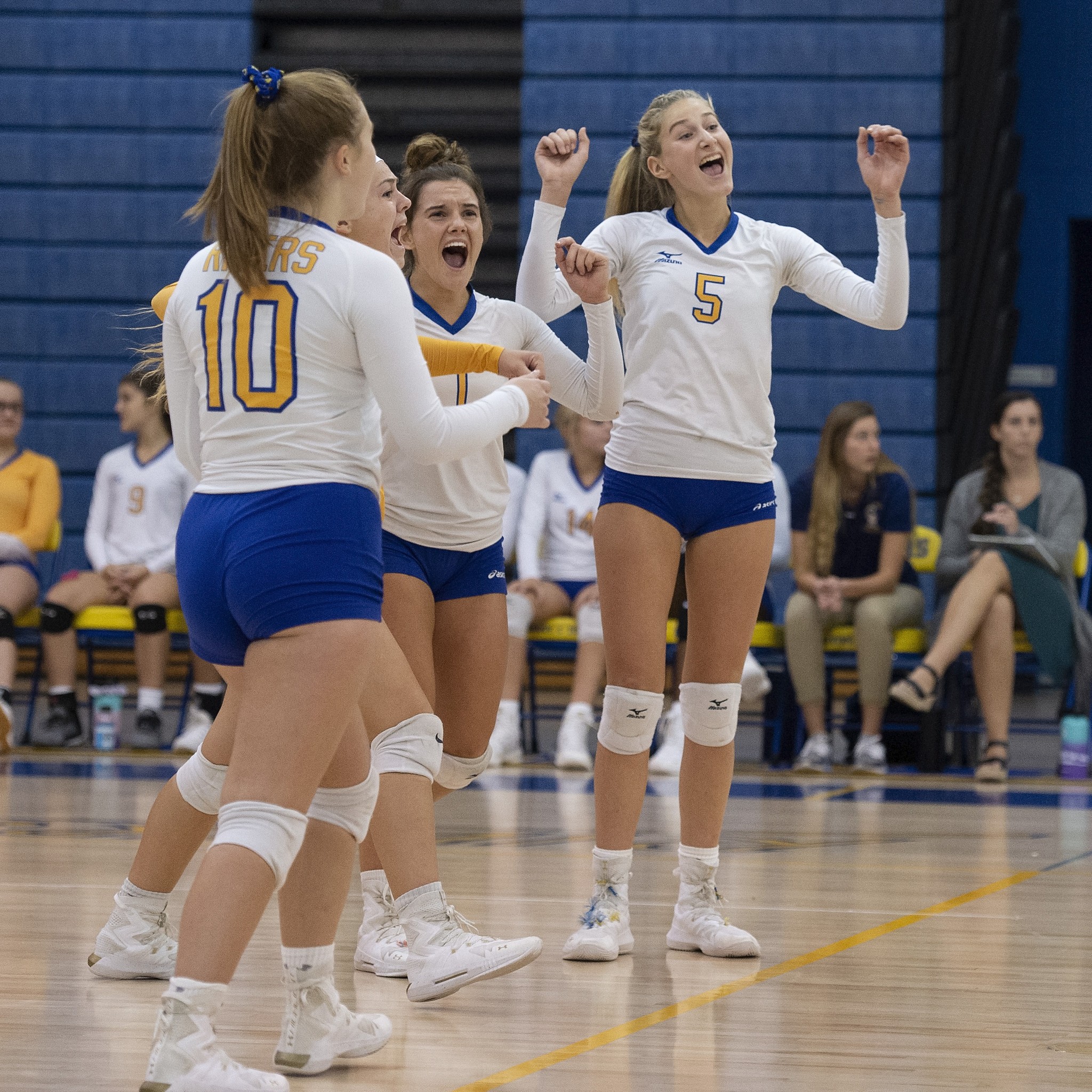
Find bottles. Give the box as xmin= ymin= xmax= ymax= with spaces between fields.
xmin=1056 ymin=700 xmax=1091 ymax=779
xmin=89 ymin=672 xmax=129 ymax=752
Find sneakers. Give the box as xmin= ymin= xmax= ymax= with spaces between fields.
xmin=485 ymin=709 xmax=524 ymax=766
xmin=33 ymin=690 xmax=84 ymax=748
xmin=139 ymin=978 xmax=289 ymax=1092
xmin=666 ymin=858 xmax=763 ymax=957
xmin=564 ymin=953 xmax=633 ymax=993
xmin=87 ymin=888 xmax=180 ymax=981
xmin=851 ymin=734 xmax=888 ymax=777
xmin=647 ymin=708 xmax=685 ymax=775
xmin=562 ymin=850 xmax=635 ymax=961
xmin=273 ymin=957 xmax=392 ymax=1073
xmin=793 ymin=732 xmax=834 ymax=774
xmin=665 ymin=949 xmax=761 ymax=993
xmin=394 ymin=890 xmax=544 ymax=1002
xmin=352 ymin=878 xmax=409 ymax=979
xmin=553 ymin=709 xmax=596 ymax=771
xmin=171 ymin=704 xmax=213 ymax=754
xmin=132 ymin=708 xmax=162 ymax=748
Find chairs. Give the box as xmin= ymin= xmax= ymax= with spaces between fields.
xmin=13 ymin=531 xmax=1092 ymax=756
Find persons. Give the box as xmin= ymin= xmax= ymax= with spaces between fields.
xmin=890 ymin=390 xmax=1092 ymax=781
xmin=89 ymin=149 xmax=545 ymax=1000
xmin=0 ymin=380 xmax=63 ymax=755
xmin=520 ymin=85 xmax=913 ymax=964
xmin=786 ymin=405 xmax=928 ymax=771
xmin=135 ymin=69 xmax=552 ymax=1092
xmin=168 ymin=660 xmax=226 ymax=755
xmin=354 ymin=137 xmax=627 ymax=979
xmin=492 ymin=407 xmax=794 ymax=773
xmin=34 ymin=378 xmax=197 ymax=747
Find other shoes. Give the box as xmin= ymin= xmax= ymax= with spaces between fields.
xmin=0 ymin=688 xmax=14 ymax=753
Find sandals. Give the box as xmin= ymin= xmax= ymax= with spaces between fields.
xmin=974 ymin=739 xmax=1012 ymax=782
xmin=889 ymin=661 xmax=940 ymax=713
